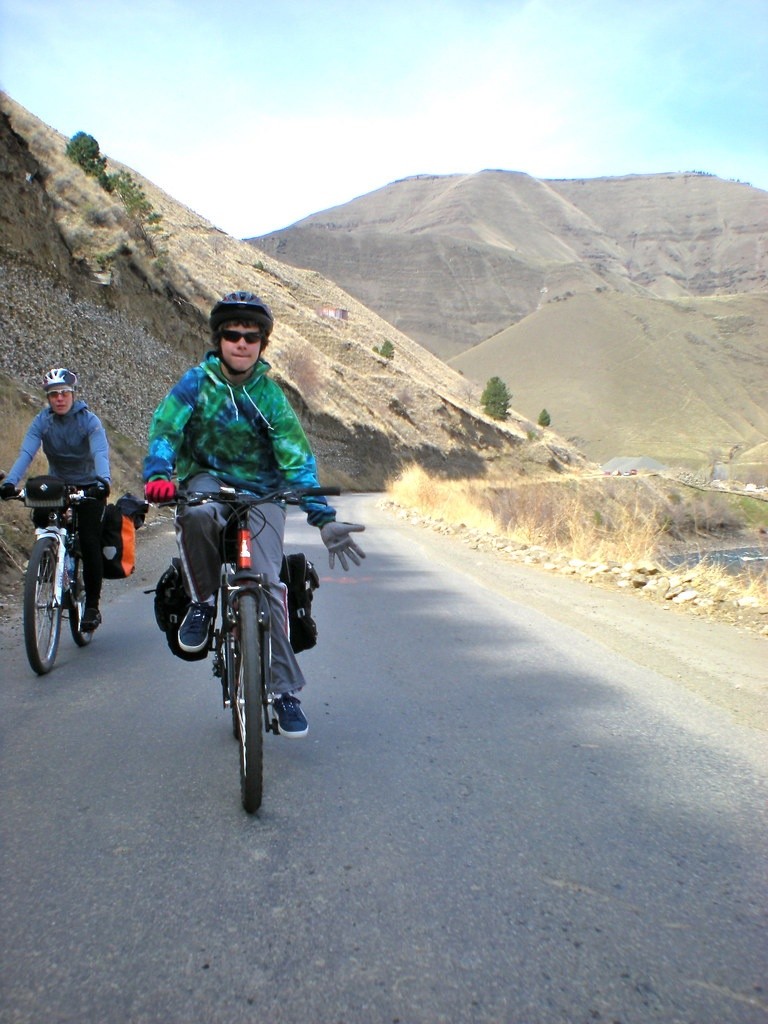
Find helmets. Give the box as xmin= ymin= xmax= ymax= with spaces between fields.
xmin=209 ymin=291 xmax=274 ymax=327
xmin=41 ymin=368 xmax=77 ymax=389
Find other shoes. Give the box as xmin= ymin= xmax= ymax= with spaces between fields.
xmin=80 ymin=607 xmax=102 ymax=632
xmin=38 ymin=569 xmax=57 ymax=583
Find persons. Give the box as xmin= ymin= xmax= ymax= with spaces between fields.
xmin=0 ymin=367 xmax=111 ymax=631
xmin=142 ymin=291 xmax=366 ymax=738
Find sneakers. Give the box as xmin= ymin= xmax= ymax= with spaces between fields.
xmin=177 ymin=602 xmax=215 ymax=652
xmin=272 ymin=692 xmax=309 ymax=740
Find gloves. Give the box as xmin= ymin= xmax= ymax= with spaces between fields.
xmin=321 ymin=520 xmax=366 ymax=571
xmin=143 ymin=478 xmax=176 ymax=504
xmin=0 ymin=482 xmax=16 ymax=498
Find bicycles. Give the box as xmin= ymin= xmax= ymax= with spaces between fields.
xmin=155 ymin=484 xmax=342 ymax=813
xmin=0 ymin=470 xmax=112 ymax=676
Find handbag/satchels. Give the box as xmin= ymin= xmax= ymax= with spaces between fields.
xmin=98 ymin=493 xmax=151 ymax=579
xmin=278 ymin=550 xmax=318 ymax=651
xmin=150 ymin=557 xmax=210 ymax=661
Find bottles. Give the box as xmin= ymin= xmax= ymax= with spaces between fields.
xmin=219 ymin=562 xmax=234 ymax=576
xmin=64 ymin=552 xmax=72 ymax=572
xmin=68 ymin=556 xmax=76 ymax=580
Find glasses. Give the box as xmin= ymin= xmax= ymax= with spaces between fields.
xmin=218 ymin=330 xmax=264 ymax=344
xmin=48 ymin=389 xmax=72 ymax=400
